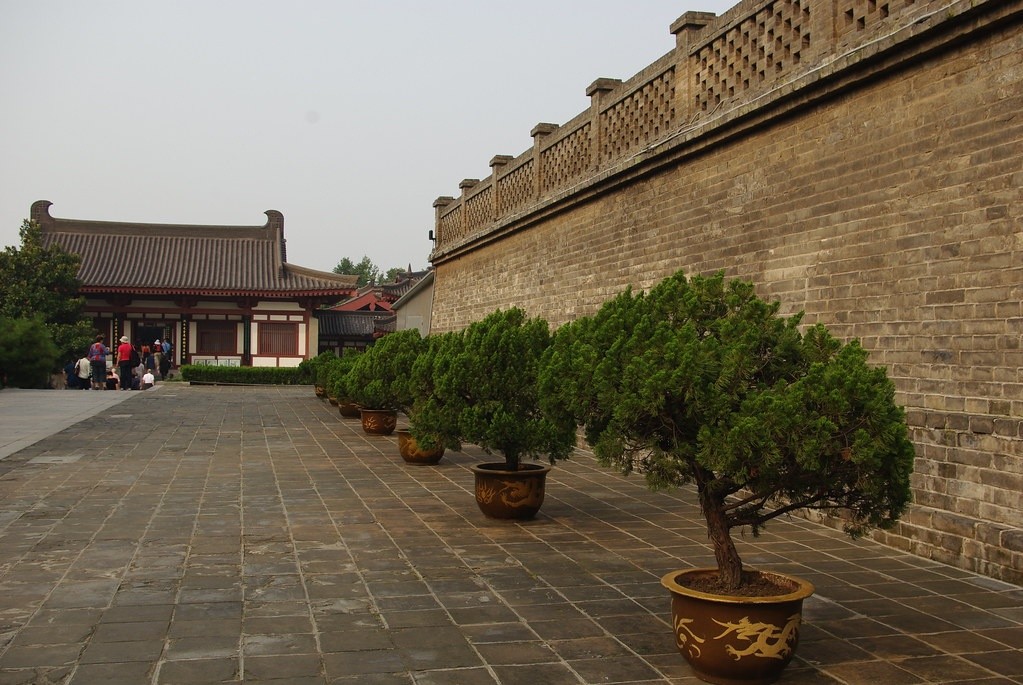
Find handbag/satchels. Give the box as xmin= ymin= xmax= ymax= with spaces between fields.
xmin=129 ymin=345 xmax=141 ymax=368
xmin=73 ymin=360 xmax=81 ymax=375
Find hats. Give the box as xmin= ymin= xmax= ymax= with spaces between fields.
xmin=154 ymin=339 xmax=160 ymax=345
xmin=119 ymin=336 xmax=129 ymax=343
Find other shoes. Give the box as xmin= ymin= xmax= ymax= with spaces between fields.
xmin=103 ymin=386 xmax=107 ymax=390
xmin=95 ymin=386 xmax=99 ymax=390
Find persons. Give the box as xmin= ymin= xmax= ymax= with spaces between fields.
xmin=63 ymin=334 xmax=173 ymax=390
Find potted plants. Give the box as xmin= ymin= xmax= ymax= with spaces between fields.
xmin=313 ymin=326 xmax=455 ymax=465
xmin=438 ymin=301 xmax=577 ymax=522
xmin=536 ymin=266 xmax=921 ymax=685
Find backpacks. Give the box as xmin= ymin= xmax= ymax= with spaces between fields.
xmin=161 ymin=354 xmax=171 ymax=372
